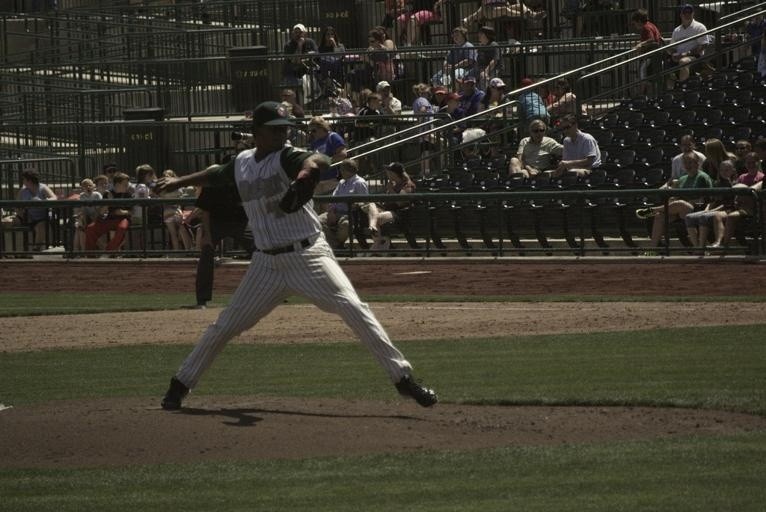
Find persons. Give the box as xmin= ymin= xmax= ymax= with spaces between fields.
xmin=150 ymin=100 xmax=441 ymax=411
xmin=1 ymin=0 xmax=765 ymax=259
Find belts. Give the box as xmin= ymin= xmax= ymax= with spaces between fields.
xmin=258 ymin=238 xmax=309 ymax=254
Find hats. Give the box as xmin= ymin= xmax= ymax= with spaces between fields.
xmin=253 ymin=101 xmax=295 ymax=127
xmin=681 ymin=5 xmax=692 ymax=14
xmin=521 ymin=78 xmax=533 ymax=87
xmin=293 ymin=24 xmax=305 ymax=32
xmin=376 ymin=81 xmax=391 ymax=93
xmin=433 ymin=86 xmax=447 ymax=95
xmin=463 ymin=76 xmax=476 ymax=83
xmin=382 ymin=162 xmax=404 ymax=174
xmin=489 ymin=78 xmax=506 ymax=87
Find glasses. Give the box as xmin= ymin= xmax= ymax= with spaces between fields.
xmin=735 ymin=148 xmax=746 ymax=150
xmin=532 ymin=130 xmax=545 ymax=132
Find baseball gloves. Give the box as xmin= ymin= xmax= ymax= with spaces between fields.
xmin=279 ymin=169 xmax=320 ymax=214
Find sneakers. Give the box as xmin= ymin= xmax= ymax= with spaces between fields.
xmin=196 ymin=300 xmax=206 ymax=310
xmin=524 ymin=10 xmax=547 ymax=23
xmin=638 ymin=250 xmax=659 ymax=256
xmin=636 ymin=207 xmax=660 ymax=219
xmin=395 ymin=375 xmax=437 ymax=407
xmin=161 ymin=376 xmax=190 ymax=409
xmin=362 ymin=226 xmax=378 ymax=235
xmin=706 ymin=243 xmax=721 ymax=248
